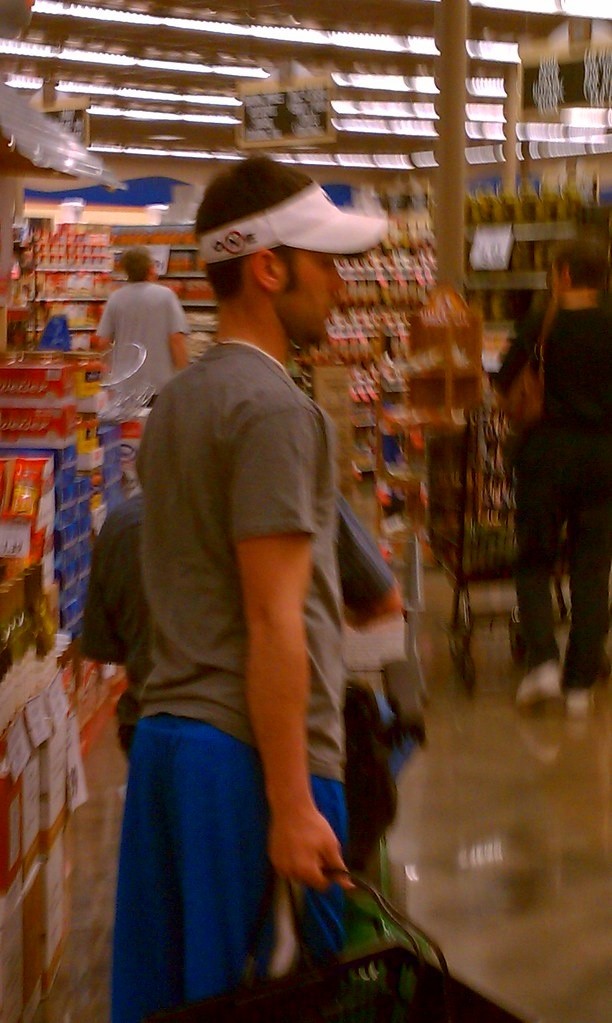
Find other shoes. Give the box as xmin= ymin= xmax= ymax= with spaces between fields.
xmin=516 ymin=660 xmax=559 ymax=707
xmin=563 ymin=686 xmax=597 ymax=716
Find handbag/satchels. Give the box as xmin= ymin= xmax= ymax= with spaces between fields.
xmin=502 ymin=290 xmax=558 ymax=429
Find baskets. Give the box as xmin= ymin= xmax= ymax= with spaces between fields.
xmin=146 ymin=861 xmax=526 ymax=1023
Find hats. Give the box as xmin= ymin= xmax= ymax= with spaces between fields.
xmin=198 ymin=178 xmax=389 ymax=264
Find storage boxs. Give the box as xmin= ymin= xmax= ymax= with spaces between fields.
xmin=0 ymin=359 xmax=158 ymax=733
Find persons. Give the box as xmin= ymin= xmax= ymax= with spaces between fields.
xmin=78 ymin=159 xmax=404 ymax=1023
xmin=494 ymin=229 xmax=612 ymax=716
xmin=94 ymin=246 xmax=191 ymax=409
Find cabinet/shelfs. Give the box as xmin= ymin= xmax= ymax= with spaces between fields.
xmin=29 ymin=172 xmax=612 ymax=575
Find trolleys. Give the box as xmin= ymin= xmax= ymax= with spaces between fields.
xmin=426 ymin=399 xmax=566 ymax=681
